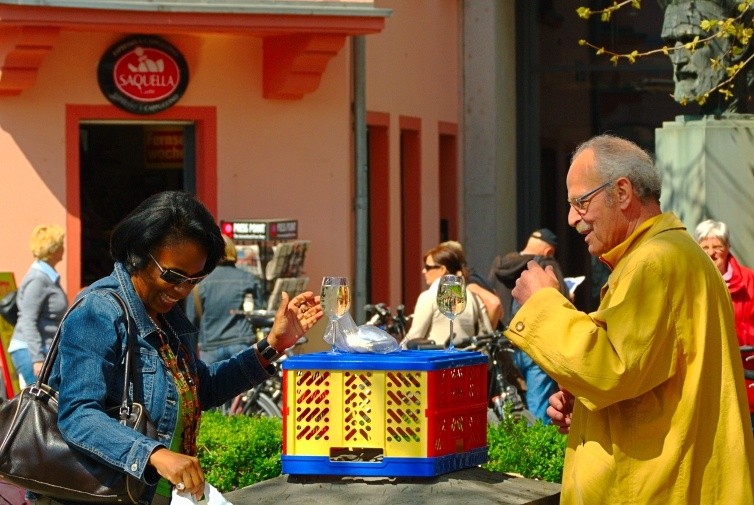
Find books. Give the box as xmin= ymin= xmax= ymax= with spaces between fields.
xmin=238 ymin=239 xmax=312 ymax=309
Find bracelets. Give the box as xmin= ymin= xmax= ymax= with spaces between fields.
xmin=257 ymin=336 xmax=283 ymax=363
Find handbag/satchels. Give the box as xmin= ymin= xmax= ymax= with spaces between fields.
xmin=0 ymin=289 xmax=160 ymax=505
xmin=0 ymin=291 xmax=19 ymax=327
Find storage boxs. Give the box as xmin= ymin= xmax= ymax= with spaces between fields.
xmin=280 ymin=348 xmax=488 ymax=477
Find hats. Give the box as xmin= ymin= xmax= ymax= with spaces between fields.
xmin=532 ymin=229 xmax=559 ymax=247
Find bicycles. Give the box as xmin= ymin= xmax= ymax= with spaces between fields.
xmin=201 ymin=302 xmax=527 ymax=439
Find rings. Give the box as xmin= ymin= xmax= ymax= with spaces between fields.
xmin=176 ymin=481 xmax=185 ymax=490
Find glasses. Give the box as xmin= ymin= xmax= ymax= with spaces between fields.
xmin=568 ymin=183 xmax=609 ymax=210
xmin=148 ymin=254 xmax=208 ymax=286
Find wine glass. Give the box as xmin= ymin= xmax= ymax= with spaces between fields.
xmin=436 ymin=274 xmax=467 ymax=354
xmin=320 ymin=276 xmax=352 ymax=356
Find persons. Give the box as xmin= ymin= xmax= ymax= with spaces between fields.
xmin=502 ymin=134 xmax=754 ymax=505
xmin=23 ymin=191 xmax=320 ymax=505
xmin=401 ymin=228 xmax=575 ymax=431
xmin=183 ymin=233 xmax=267 ymax=419
xmin=8 ymin=226 xmax=69 ymax=390
